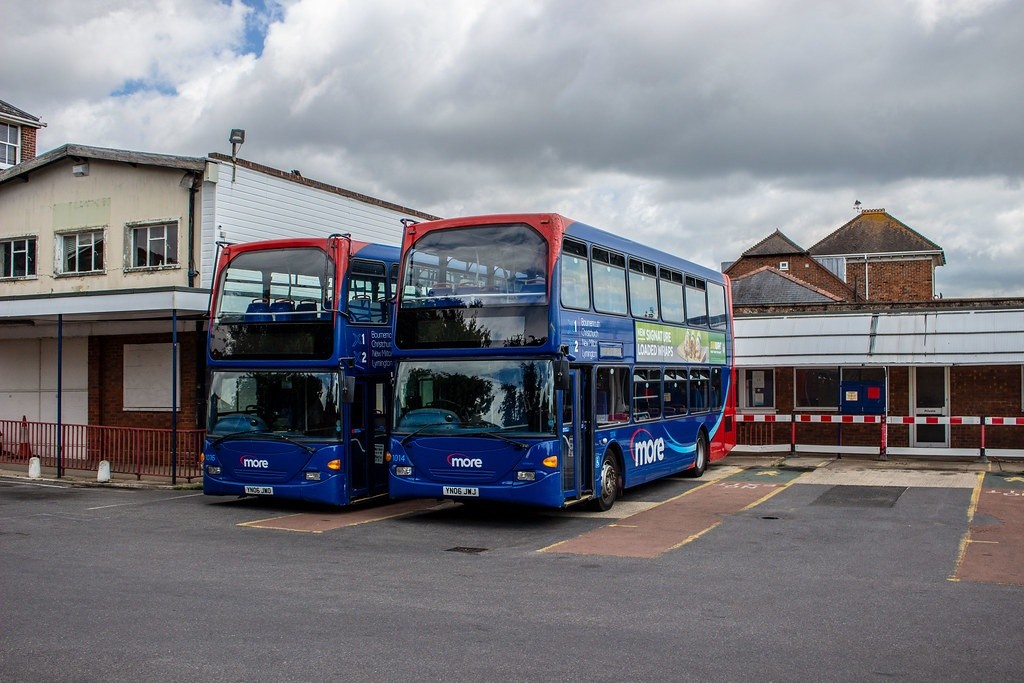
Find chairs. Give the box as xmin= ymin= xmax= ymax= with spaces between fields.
xmin=427 ymin=278 xmax=546 ymax=294
xmin=596 ymin=387 xmax=719 ymax=419
xmin=244 ymin=295 xmax=387 ymax=323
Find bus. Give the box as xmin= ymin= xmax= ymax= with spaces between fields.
xmin=197 ymin=233 xmax=543 ymax=513
xmin=379 ymin=211 xmax=737 ymax=513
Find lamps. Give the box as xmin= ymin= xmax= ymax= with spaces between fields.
xmin=228 ymin=129 xmax=245 ymax=162
